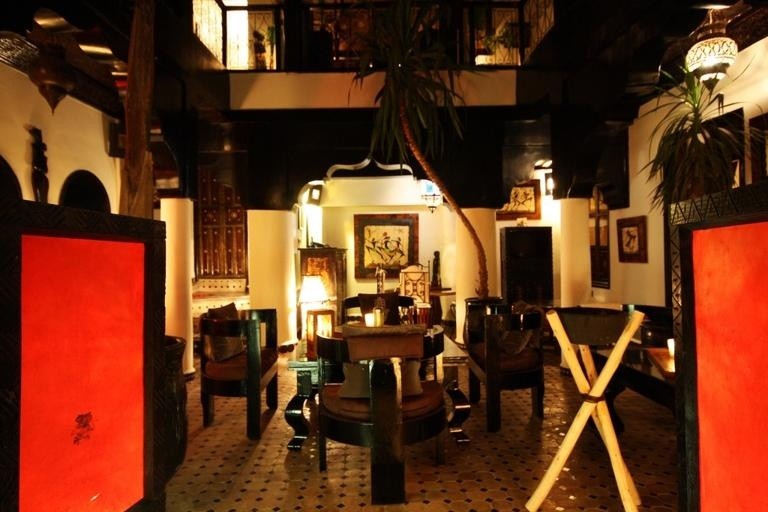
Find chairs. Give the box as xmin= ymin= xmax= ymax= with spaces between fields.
xmin=198 ymin=308 xmax=279 ymax=439
xmin=338 ymin=289 xmax=418 ymax=330
xmin=463 ymin=301 xmax=544 ymax=434
xmin=313 ymin=324 xmax=447 ymax=507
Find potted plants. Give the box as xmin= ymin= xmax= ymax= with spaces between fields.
xmin=342 ymin=0 xmax=506 ymax=359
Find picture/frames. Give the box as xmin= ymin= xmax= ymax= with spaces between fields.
xmin=495 ymin=178 xmax=541 ymax=221
xmin=354 ymin=213 xmax=419 ymax=281
xmin=615 ymin=215 xmax=648 ymax=263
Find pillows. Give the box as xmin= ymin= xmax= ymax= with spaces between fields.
xmin=336 ymin=323 xmax=424 ymax=400
xmin=356 ymin=291 xmax=404 ymax=325
xmin=204 ymin=302 xmax=245 ymax=362
xmin=493 ymin=301 xmax=538 ymax=353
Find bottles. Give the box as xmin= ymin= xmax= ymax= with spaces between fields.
xmin=152 ymin=164 xmax=162 ymax=220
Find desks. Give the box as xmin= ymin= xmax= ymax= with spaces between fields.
xmin=282 ymin=332 xmax=472 ymax=452
xmin=593 ymin=348 xmax=676 ymax=439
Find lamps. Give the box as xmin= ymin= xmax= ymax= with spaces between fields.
xmin=681 ymin=10 xmax=740 ymax=88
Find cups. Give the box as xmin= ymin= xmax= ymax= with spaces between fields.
xmin=373 ymin=307 xmax=384 ymax=327
xmin=364 ymin=313 xmax=374 ymax=327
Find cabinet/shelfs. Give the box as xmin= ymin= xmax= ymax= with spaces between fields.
xmin=296 ymin=244 xmax=348 ymax=336
